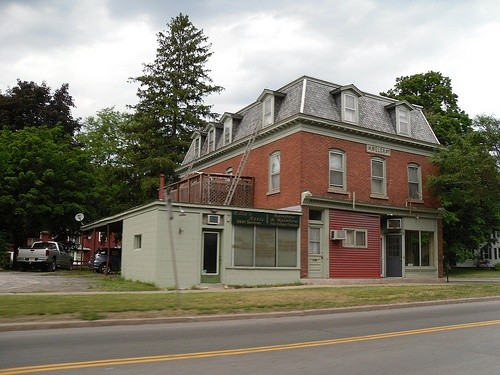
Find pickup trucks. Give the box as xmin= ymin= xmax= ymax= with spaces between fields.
xmin=14 ymin=240 xmax=74 ymax=272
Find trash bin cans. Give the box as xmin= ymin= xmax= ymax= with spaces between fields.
xmin=23 ymin=262 xmax=32 ymax=273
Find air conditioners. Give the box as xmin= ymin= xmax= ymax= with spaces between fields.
xmin=329 ymin=230 xmax=347 ymax=240
xmin=207 ymin=215 xmax=221 ymax=225
xmin=387 ymin=219 xmax=401 ymax=230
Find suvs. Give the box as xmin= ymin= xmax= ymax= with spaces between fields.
xmin=88 ymin=247 xmax=121 ymax=274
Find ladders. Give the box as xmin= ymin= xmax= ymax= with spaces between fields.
xmin=223 ymin=120 xmax=263 ymax=205
